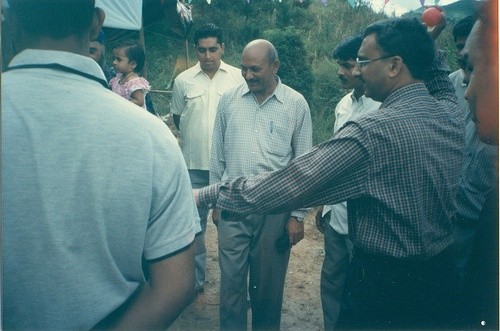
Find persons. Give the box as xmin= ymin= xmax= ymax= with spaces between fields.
xmin=462 ymin=0 xmax=499 ymax=330
xmin=89 ymin=27 xmax=155 ymax=114
xmin=209 ymin=39 xmax=313 ymax=331
xmin=108 ymin=38 xmax=151 ymax=112
xmin=170 ymin=24 xmax=248 ymax=292
xmin=316 ymin=34 xmax=382 ymax=331
xmin=193 ymin=4 xmax=467 ymax=331
xmin=447 ymin=15 xmax=477 ymax=144
xmin=0 ymin=1 xmax=204 ymax=330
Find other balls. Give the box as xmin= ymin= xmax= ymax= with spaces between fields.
xmin=422 ymin=7 xmax=441 ymax=28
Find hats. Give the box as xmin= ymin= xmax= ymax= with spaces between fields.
xmin=95 ymin=29 xmax=104 ymax=44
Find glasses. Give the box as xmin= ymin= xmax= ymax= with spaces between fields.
xmin=356 ymin=58 xmax=385 ymax=70
